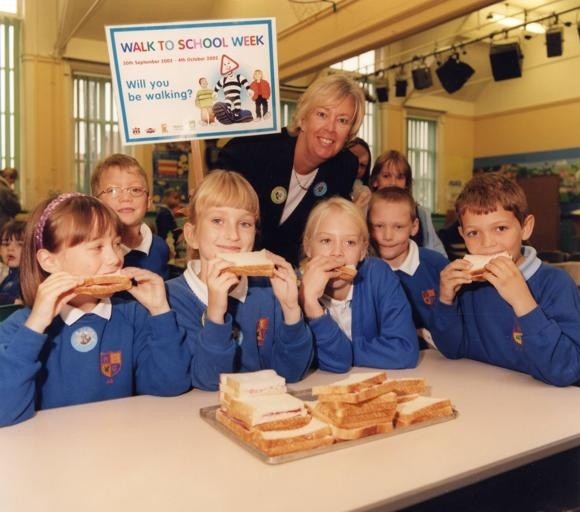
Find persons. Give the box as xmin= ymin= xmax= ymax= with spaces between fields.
xmin=432 ymin=171 xmax=579 ymax=388
xmin=155 ymin=189 xmax=184 ymax=260
xmin=88 ymin=153 xmax=171 ymax=279
xmin=1 ymin=165 xmax=25 ymax=229
xmin=0 ymin=222 xmax=30 ymax=313
xmin=366 ymin=149 xmax=449 ymax=260
xmin=354 ymin=185 xmax=451 ymax=352
xmin=292 ymin=197 xmax=419 ymax=372
xmin=0 ymin=192 xmax=192 ymax=426
xmin=346 ymin=136 xmax=373 ymax=219
xmin=216 ymin=68 xmax=365 ymax=281
xmin=161 ymin=169 xmax=313 ymax=395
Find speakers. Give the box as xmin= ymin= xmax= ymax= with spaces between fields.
xmin=374 ymin=26 xmax=565 ymax=104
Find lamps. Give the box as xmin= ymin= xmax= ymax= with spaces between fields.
xmin=353 ymin=7 xmax=579 ymax=104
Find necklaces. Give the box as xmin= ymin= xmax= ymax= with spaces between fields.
xmin=292 ymin=163 xmax=314 ymax=192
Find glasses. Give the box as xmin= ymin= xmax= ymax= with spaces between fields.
xmin=96 ymin=185 xmax=150 ymax=199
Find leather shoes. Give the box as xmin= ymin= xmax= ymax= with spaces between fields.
xmin=229 ymin=109 xmax=253 ymax=123
xmin=211 ymin=101 xmax=235 ymax=124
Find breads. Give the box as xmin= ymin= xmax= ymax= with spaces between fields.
xmin=462 ymin=250 xmax=513 ymax=283
xmin=215 ymin=369 xmax=454 ymax=456
xmin=216 ymin=251 xmax=276 ymax=278
xmin=334 ymin=265 xmax=358 ymax=283
xmin=72 ymin=272 xmax=134 ymax=296
